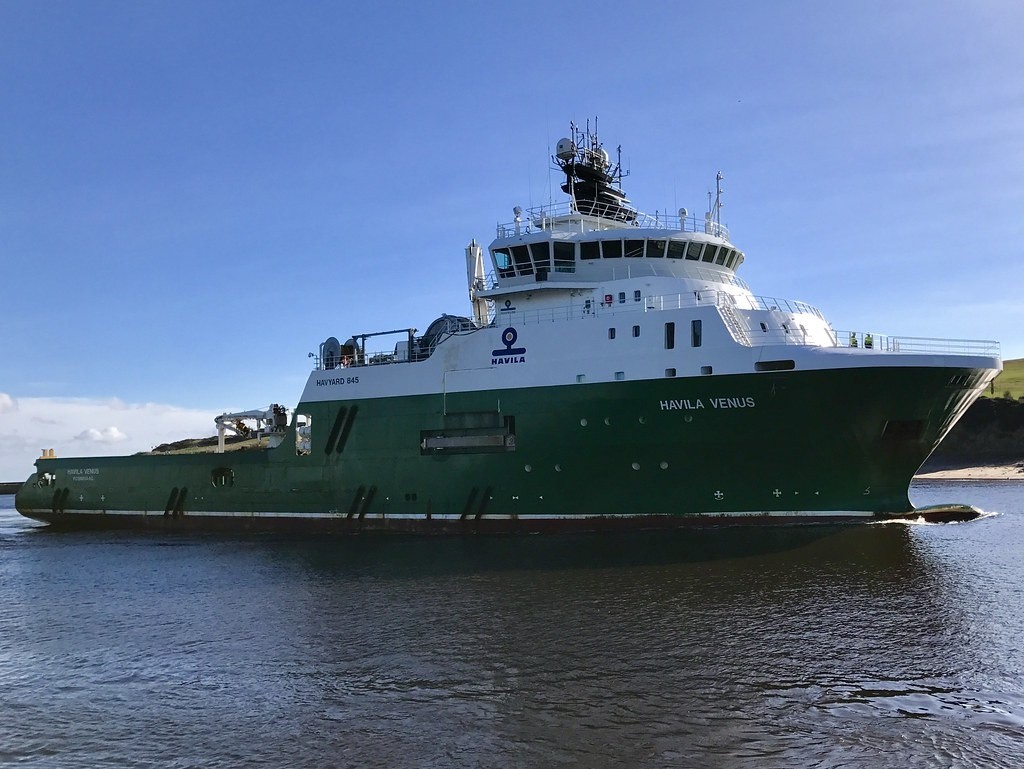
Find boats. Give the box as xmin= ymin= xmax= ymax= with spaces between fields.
xmin=13 ymin=113 xmax=1002 ymax=533
